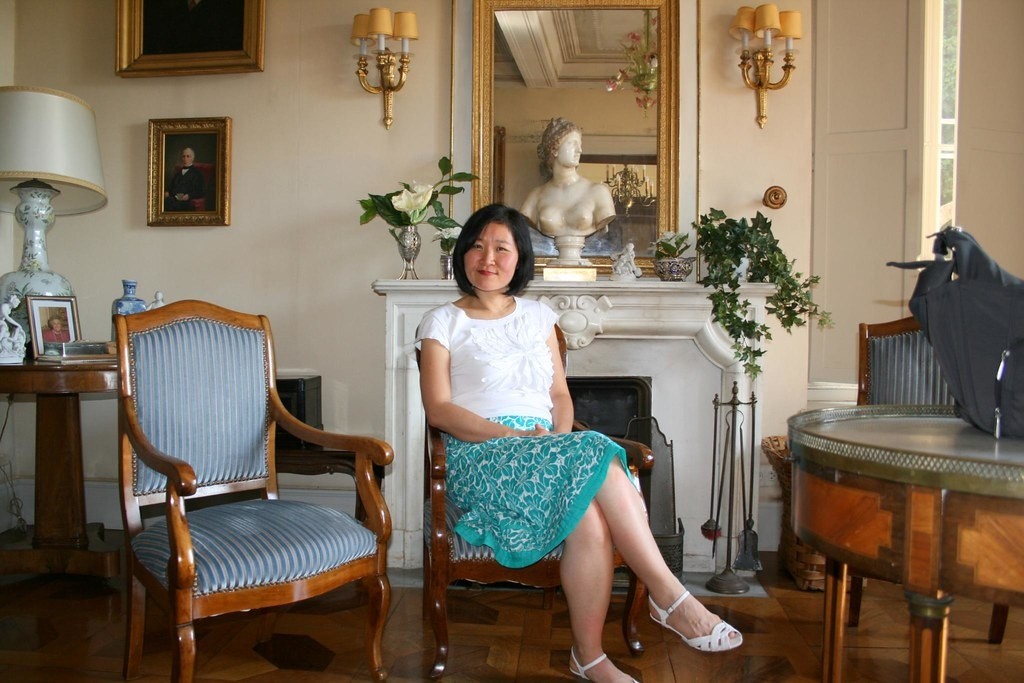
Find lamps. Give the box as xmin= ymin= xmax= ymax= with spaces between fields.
xmin=730 ymin=4 xmax=803 ymax=130
xmin=0 ymin=84 xmax=108 ymax=349
xmin=350 ymin=7 xmax=418 ymax=130
xmin=604 ymin=9 xmax=658 ymax=112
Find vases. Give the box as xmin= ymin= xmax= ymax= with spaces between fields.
xmin=111 ymin=279 xmax=147 ymax=342
xmin=652 ymin=257 xmax=697 ymax=280
xmin=399 ymin=226 xmax=457 ymax=279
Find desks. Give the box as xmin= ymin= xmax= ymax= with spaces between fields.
xmin=0 ymin=359 xmax=123 ymax=583
xmin=786 ymin=404 xmax=1024 ymax=683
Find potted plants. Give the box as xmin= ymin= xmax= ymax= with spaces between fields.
xmin=693 ymin=207 xmax=835 ymax=380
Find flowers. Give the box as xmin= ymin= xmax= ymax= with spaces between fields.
xmin=648 ymin=231 xmax=691 ymax=261
xmin=358 ymin=158 xmax=481 ymax=253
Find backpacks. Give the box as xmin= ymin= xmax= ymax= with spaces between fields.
xmin=885 ymin=226 xmax=1024 ymax=443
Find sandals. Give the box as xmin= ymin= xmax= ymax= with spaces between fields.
xmin=568 ymin=645 xmax=639 ymax=683
xmin=647 ymin=589 xmax=744 ymax=652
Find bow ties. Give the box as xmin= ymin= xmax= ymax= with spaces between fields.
xmin=182 ymin=166 xmax=190 ymax=169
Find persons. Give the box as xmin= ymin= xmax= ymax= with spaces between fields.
xmin=0 ymin=294 xmax=22 ymax=340
xmin=519 ymin=118 xmax=616 ymax=238
xmin=612 ymin=243 xmax=636 ymax=275
xmin=42 ymin=316 xmax=70 ymax=342
xmin=414 ymin=203 xmax=743 ymax=683
xmin=145 ymin=290 xmax=165 ymax=311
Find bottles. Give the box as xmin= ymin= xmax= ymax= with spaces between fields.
xmin=111 ymin=279 xmax=145 ymax=341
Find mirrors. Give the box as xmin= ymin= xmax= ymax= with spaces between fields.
xmin=470 ymin=0 xmax=682 ymax=275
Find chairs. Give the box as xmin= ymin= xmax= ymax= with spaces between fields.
xmin=414 ymin=323 xmax=658 ymax=679
xmin=845 ymin=316 xmax=1009 ymax=643
xmin=108 ymin=299 xmax=397 ymax=683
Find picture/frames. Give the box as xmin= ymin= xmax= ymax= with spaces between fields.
xmin=26 ymin=294 xmax=82 ymax=360
xmin=147 ymin=116 xmax=231 ymax=226
xmin=114 ymin=0 xmax=267 ymax=78
